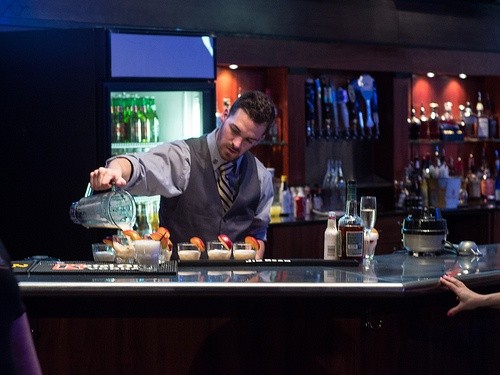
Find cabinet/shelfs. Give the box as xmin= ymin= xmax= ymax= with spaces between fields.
xmin=215 ymin=66 xmax=499 ymax=259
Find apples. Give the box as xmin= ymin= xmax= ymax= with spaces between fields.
xmin=104 ymin=226 xmax=172 ymax=251
xmin=190 ymin=237 xmax=205 ymax=251
xmin=217 ymin=234 xmax=232 ymax=250
xmin=245 ymin=236 xmax=260 ymax=251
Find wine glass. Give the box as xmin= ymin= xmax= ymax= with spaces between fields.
xmin=360 ymin=196 xmax=377 ymax=266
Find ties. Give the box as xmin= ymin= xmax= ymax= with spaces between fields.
xmin=217 ymin=162 xmax=236 ymax=214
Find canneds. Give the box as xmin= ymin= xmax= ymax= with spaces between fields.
xmin=295 ymin=196 xmax=307 ymax=219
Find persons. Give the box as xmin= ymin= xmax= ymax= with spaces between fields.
xmin=0 ymin=264 xmax=42 ymax=375
xmin=91 ymin=92 xmax=275 ymax=260
xmin=440 ymin=275 xmax=500 ymax=316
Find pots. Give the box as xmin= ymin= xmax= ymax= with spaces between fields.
xmin=401 ymin=208 xmax=447 ymax=256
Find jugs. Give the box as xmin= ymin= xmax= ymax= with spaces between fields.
xmin=70 ymin=181 xmax=136 ymax=233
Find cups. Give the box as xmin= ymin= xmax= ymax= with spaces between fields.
xmin=134 ymin=240 xmax=161 ymax=271
xmin=92 ymin=244 xmax=115 ymax=262
xmin=112 ymin=235 xmax=134 ymax=264
xmin=207 ymin=241 xmax=231 ymax=260
xmin=178 ymin=244 xmax=201 ymax=261
xmin=232 ymin=243 xmax=256 ymax=260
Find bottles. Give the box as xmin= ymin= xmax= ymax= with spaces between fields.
xmin=111 ymin=94 xmax=161 ymax=142
xmin=409 ymin=144 xmax=500 ymax=206
xmin=410 ymin=91 xmax=500 ymax=141
xmin=133 ymin=203 xmax=150 ymax=239
xmin=264 ymin=167 xmax=323 ymax=219
xmin=223 ymin=87 xmax=277 ymax=144
xmin=323 ymin=212 xmax=338 ymax=260
xmin=305 ymin=74 xmax=381 ymax=140
xmin=338 ymin=179 xmax=364 ymax=266
xmin=151 ymin=201 xmax=159 ymax=233
xmin=323 ymin=158 xmax=346 ymax=217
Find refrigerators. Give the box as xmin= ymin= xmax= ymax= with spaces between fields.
xmin=0 ymin=28 xmax=216 ymax=262
